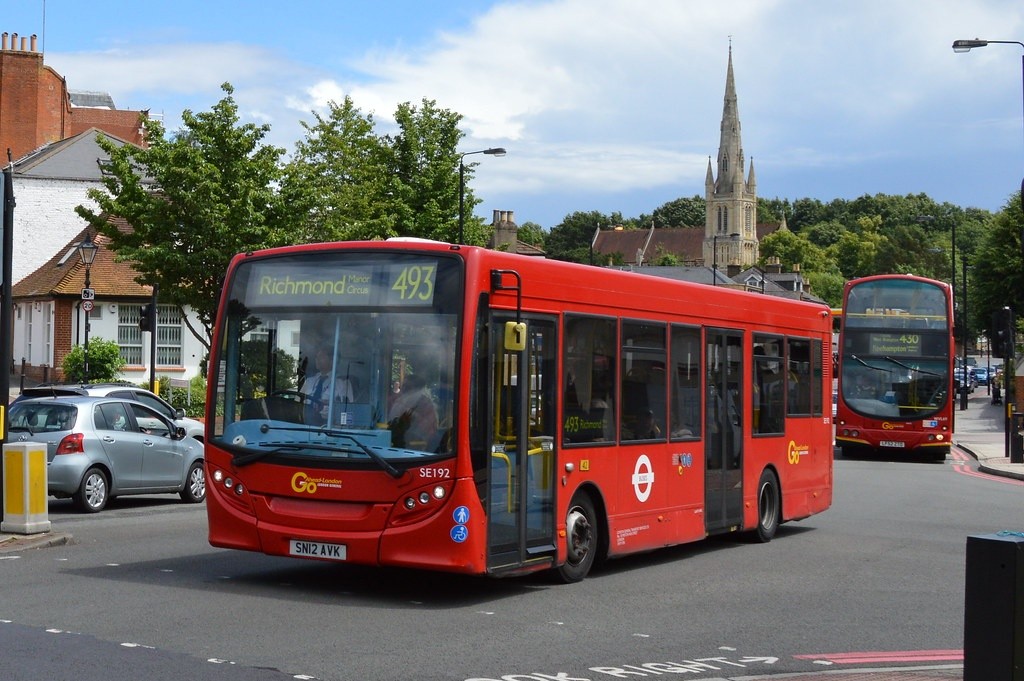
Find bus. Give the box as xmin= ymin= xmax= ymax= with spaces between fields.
xmin=201 ymin=232 xmax=835 ymax=589
xmin=828 ymin=306 xmax=848 ymax=420
xmin=833 ymin=274 xmax=956 ymax=461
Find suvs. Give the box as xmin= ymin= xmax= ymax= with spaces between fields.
xmin=8 ymin=380 xmax=205 ymax=446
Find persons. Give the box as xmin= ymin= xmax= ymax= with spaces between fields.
xmin=390 ymin=375 xmax=437 ymax=442
xmin=850 ymin=375 xmax=876 ymax=399
xmin=294 ymin=345 xmax=354 ymax=422
xmin=991 ymin=378 xmax=1000 ymax=406
xmin=391 ymin=380 xmax=400 ymax=401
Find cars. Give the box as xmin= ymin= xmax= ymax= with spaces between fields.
xmin=8 ymin=394 xmax=206 ymax=514
xmin=954 ymin=365 xmax=1005 ymax=394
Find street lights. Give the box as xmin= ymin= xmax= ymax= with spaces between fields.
xmin=76 ymin=231 xmax=99 ymax=383
xmin=1001 ymin=305 xmax=1012 ymax=458
xmin=712 ymin=233 xmax=741 ymax=285
xmin=457 ymin=148 xmax=506 ymax=244
